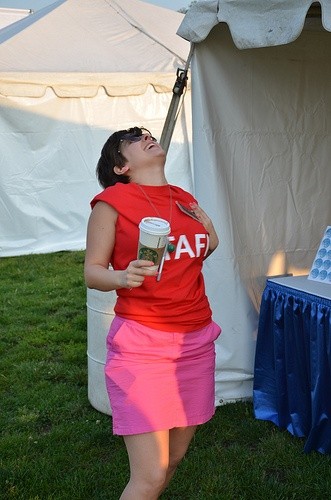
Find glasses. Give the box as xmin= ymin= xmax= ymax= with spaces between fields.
xmin=118 ymin=134 xmax=157 ymax=153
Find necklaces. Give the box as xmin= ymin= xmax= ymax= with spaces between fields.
xmin=134 ymin=181 xmax=173 ymax=228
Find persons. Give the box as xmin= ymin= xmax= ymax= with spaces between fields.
xmin=84 ymin=127 xmax=223 ymax=500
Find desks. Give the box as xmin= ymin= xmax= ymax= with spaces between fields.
xmin=252 ymin=275 xmax=331 ymax=453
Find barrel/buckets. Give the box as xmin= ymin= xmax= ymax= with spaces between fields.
xmin=86 ymin=266 xmax=118 ymax=417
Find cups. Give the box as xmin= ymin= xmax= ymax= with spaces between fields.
xmin=138 ymin=216 xmax=171 ymax=273
